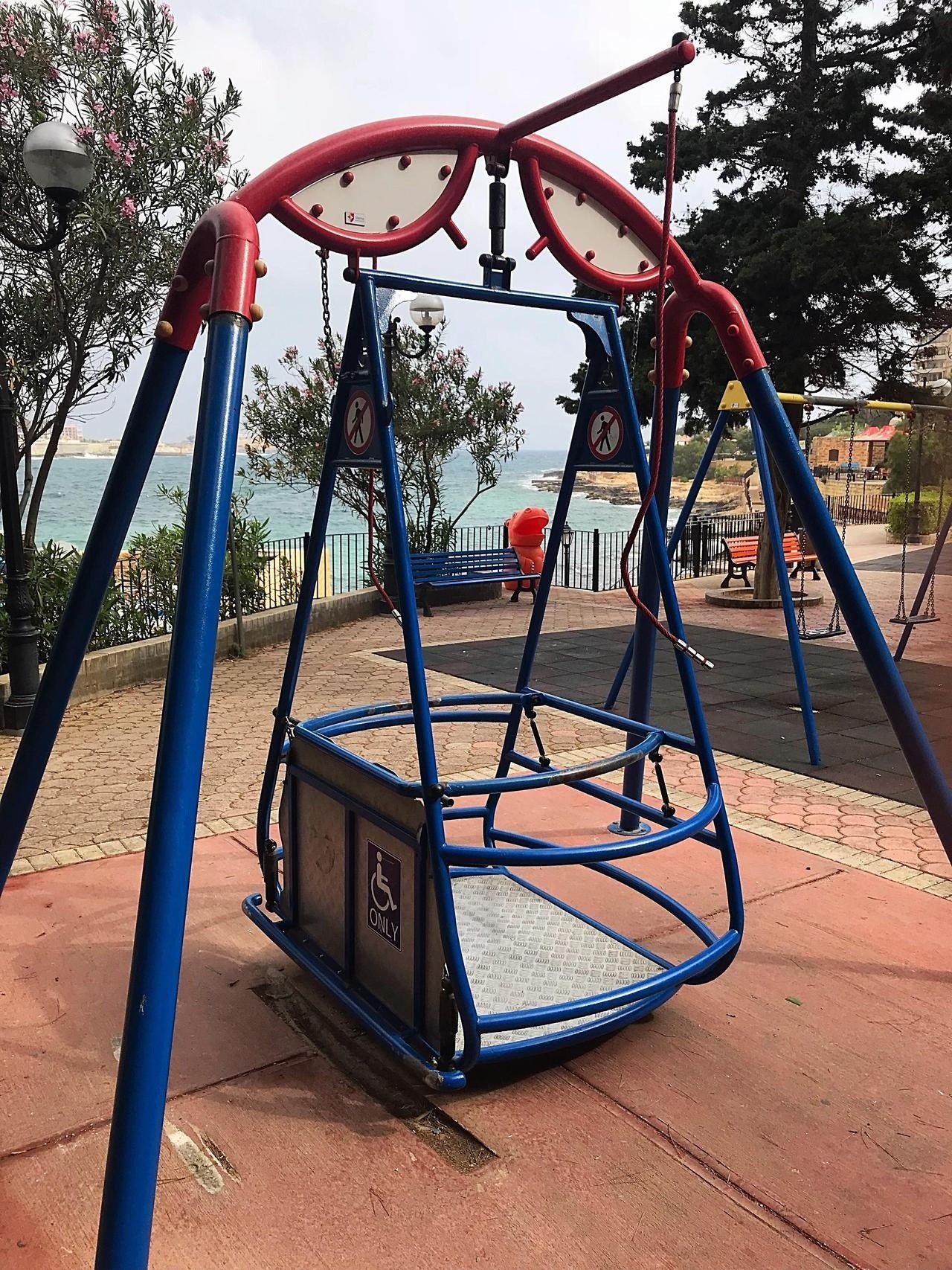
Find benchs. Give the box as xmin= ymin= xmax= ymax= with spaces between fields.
xmin=838 ymin=468 xmax=892 ymax=480
xmin=720 ymin=532 xmax=821 ymax=588
xmin=408 ymin=547 xmax=541 ymax=617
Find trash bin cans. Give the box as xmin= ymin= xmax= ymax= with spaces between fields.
xmin=840 ymin=461 xmax=859 ymax=468
xmin=504 ymin=508 xmax=550 ymax=590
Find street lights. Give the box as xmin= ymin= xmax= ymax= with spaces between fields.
xmin=903 ymin=376 xmax=952 ymax=544
xmin=0 ymin=122 xmax=97 ymax=735
xmin=381 ymin=293 xmax=445 ymax=404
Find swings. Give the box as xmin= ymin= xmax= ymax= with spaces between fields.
xmin=889 ymin=416 xmax=950 ymax=626
xmin=796 ymin=410 xmax=856 ymax=640
